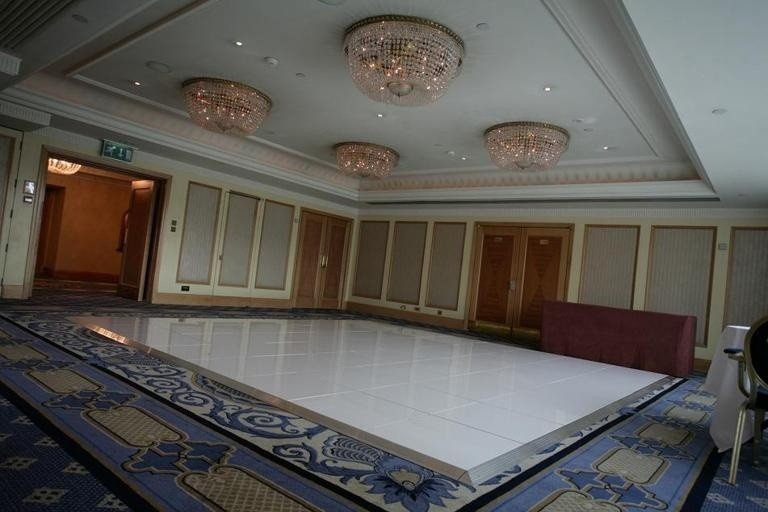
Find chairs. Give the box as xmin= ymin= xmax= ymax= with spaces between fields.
xmin=724 ymin=316 xmax=767 ymax=484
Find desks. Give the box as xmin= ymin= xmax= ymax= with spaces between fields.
xmin=713 ymin=324 xmax=758 ymax=453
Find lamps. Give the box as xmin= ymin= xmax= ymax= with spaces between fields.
xmin=332 ymin=140 xmax=401 ymax=183
xmin=341 ymin=15 xmax=466 ymax=109
xmin=180 ymin=76 xmax=275 ymax=137
xmin=484 ymin=120 xmax=571 ymax=174
